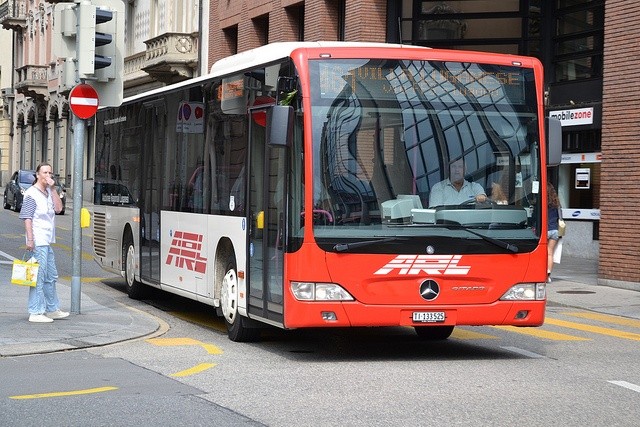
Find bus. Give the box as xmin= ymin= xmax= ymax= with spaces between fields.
xmin=93 ymin=40 xmax=550 ymax=342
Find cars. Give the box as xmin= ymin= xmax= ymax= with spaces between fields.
xmin=4 ymin=170 xmax=66 ymax=215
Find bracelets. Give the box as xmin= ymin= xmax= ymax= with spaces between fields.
xmin=28 ymin=238 xmax=33 ymax=241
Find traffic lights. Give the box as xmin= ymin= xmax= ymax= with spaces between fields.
xmin=76 ymin=5 xmax=112 ymax=80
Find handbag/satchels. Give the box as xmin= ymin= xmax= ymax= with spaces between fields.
xmin=558 ymin=219 xmax=566 ymax=236
xmin=552 ymin=239 xmax=563 ymax=264
xmin=11 ymin=259 xmax=39 ymax=287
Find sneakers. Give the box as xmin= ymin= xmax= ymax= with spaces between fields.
xmin=547 ymin=278 xmax=551 ymax=282
xmin=28 ymin=314 xmax=53 ymax=323
xmin=45 ymin=311 xmax=70 ymax=320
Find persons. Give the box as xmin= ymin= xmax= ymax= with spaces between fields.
xmin=428 ymin=152 xmax=487 ymax=210
xmin=19 ymin=162 xmax=70 ymax=323
xmin=536 ymin=181 xmax=563 ymax=283
xmin=488 ymin=181 xmax=508 ymax=205
xmin=521 ymin=177 xmax=537 ymax=228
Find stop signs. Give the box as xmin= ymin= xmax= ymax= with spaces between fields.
xmin=68 ymin=84 xmax=99 ymax=119
